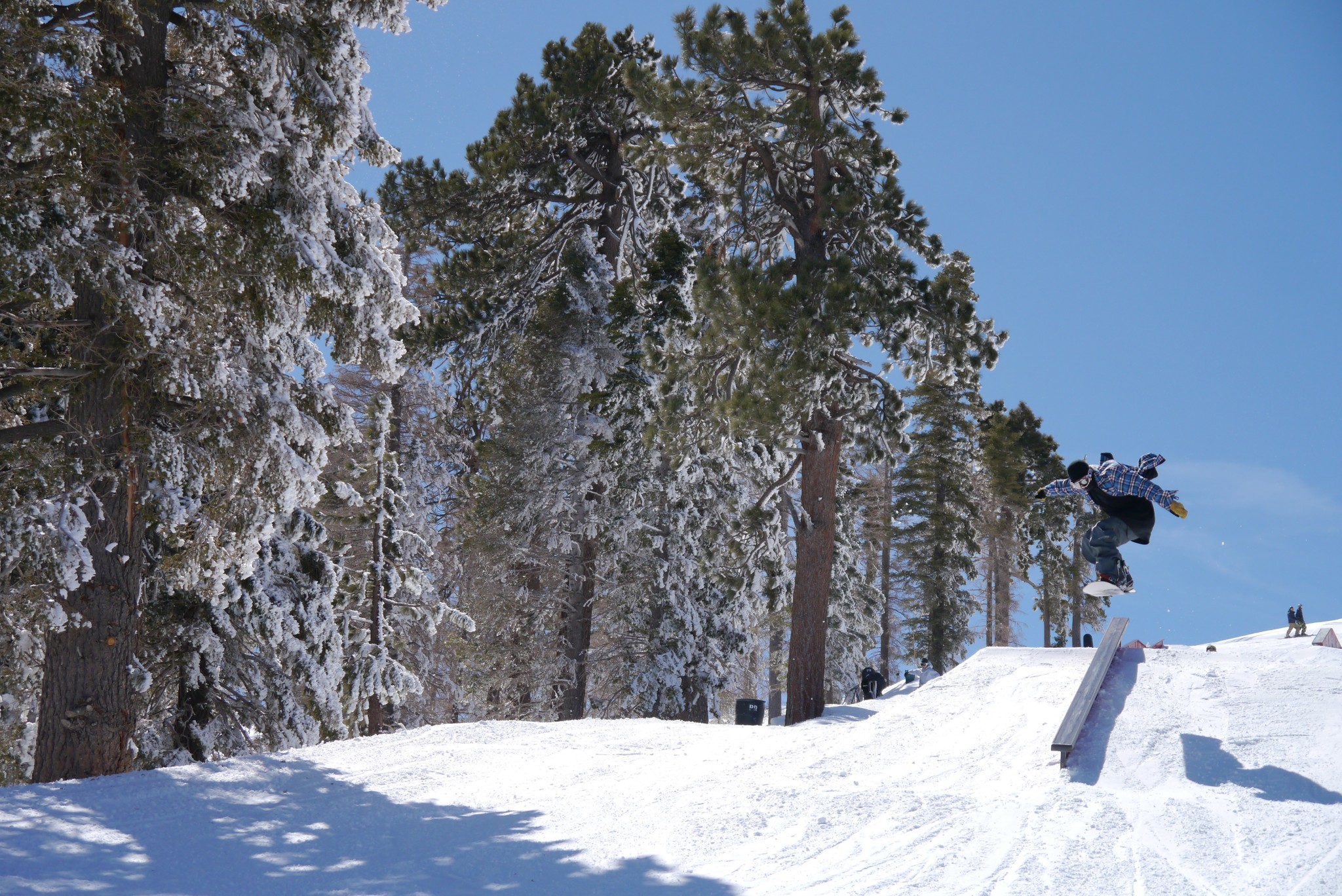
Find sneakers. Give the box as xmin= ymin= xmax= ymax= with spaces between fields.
xmin=1295 ymin=634 xmax=1301 ymax=636
xmin=1099 ymin=560 xmax=1134 ymax=592
xmin=1301 ymin=634 xmax=1308 ymax=636
xmin=1286 ymin=636 xmax=1291 ymax=638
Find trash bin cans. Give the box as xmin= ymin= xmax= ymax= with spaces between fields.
xmin=735 ymin=698 xmax=764 ymax=726
xmin=905 ymin=670 xmax=914 ymax=683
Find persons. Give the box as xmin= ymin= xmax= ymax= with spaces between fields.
xmin=861 ymin=667 xmax=887 ymax=701
xmin=1286 ymin=604 xmax=1308 ymax=638
xmin=1206 ymin=645 xmax=1216 ymax=652
xmin=905 ymin=658 xmax=940 ymax=687
xmin=1034 ymin=452 xmax=1188 ymax=592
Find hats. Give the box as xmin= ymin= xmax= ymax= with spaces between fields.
xmin=1067 ymin=459 xmax=1089 ymax=483
xmin=1298 ymin=604 xmax=1303 ymax=609
xmin=921 ymin=658 xmax=929 ymax=663
xmin=1290 ymin=606 xmax=1294 ymax=610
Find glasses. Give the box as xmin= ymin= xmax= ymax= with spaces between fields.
xmin=920 ymin=663 xmax=928 ymax=666
xmin=1071 ymin=477 xmax=1090 ymax=488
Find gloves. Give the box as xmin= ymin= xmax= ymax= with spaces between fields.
xmin=1170 ymin=500 xmax=1188 ymax=519
xmin=1035 ymin=488 xmax=1046 ymax=501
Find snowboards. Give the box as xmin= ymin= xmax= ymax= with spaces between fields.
xmin=1082 ymin=581 xmax=1137 ymax=597
